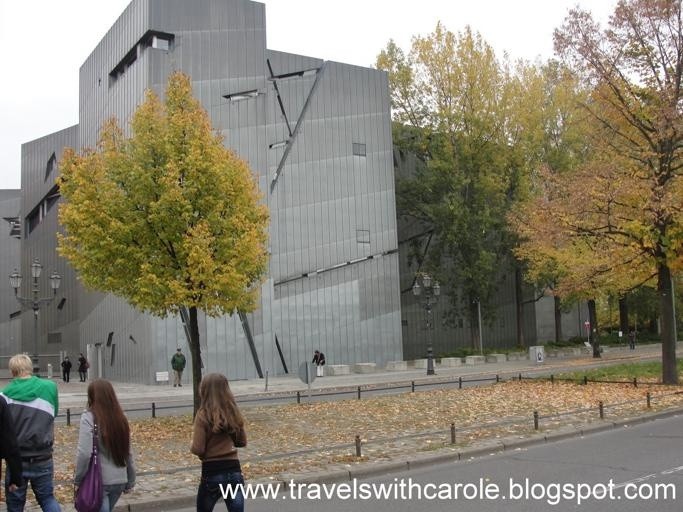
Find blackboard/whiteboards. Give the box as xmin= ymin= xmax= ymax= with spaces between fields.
xmin=155 ymin=371 xmax=168 ymax=381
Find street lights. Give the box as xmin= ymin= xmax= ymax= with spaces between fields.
xmin=9 ymin=259 xmax=61 ymax=379
xmin=413 ymin=273 xmax=440 ymax=375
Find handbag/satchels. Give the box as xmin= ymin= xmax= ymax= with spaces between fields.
xmin=75 ymin=453 xmax=102 ymax=512
xmin=84 ymin=362 xmax=90 ymax=369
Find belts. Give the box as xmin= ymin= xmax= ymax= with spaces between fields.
xmin=19 ymin=455 xmax=52 ymax=464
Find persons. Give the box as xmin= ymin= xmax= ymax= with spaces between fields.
xmin=188 ymin=372 xmax=250 ymax=512
xmin=0 ymin=351 xmax=64 ymax=512
xmin=60 ymin=356 xmax=72 ymax=384
xmin=76 ymin=352 xmax=88 ymax=383
xmin=169 ymin=348 xmax=186 ymax=388
xmin=72 ymin=377 xmax=137 ymax=512
xmin=311 ymin=349 xmax=326 ymax=378
xmin=590 ymin=329 xmax=601 ymax=359
xmin=0 ymin=393 xmax=24 ymax=494
xmin=627 ymin=331 xmax=636 ymax=349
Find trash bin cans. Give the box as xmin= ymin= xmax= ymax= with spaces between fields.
xmin=529 ymin=346 xmax=544 ymax=364
